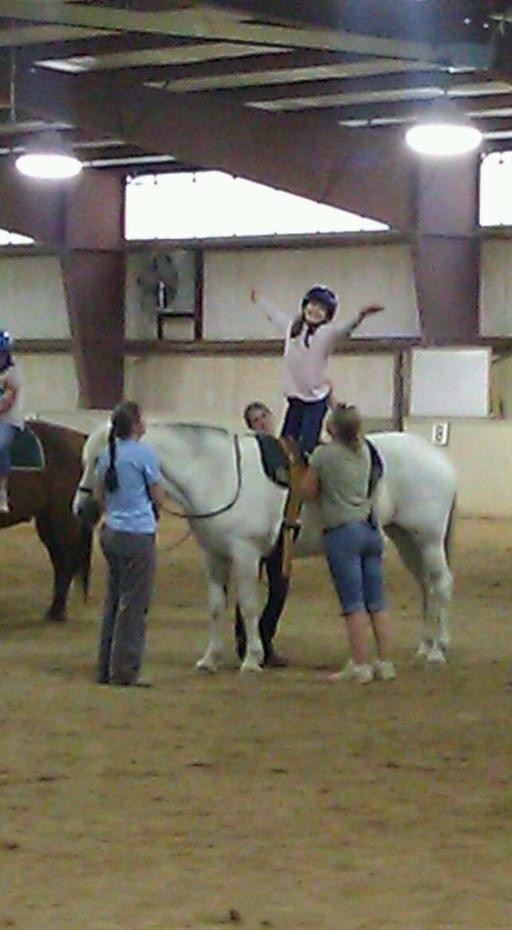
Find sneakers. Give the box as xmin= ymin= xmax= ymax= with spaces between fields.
xmin=0 ymin=489 xmax=10 ymax=512
xmin=97 ymin=674 xmax=154 ymax=690
xmin=326 ymin=659 xmax=373 ymax=685
xmin=264 ymin=640 xmax=290 ymax=668
xmin=368 ymin=658 xmax=396 ymax=682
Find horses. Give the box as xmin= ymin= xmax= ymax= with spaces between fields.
xmin=72 ymin=411 xmax=460 ymax=678
xmin=0 ymin=419 xmax=94 ymax=623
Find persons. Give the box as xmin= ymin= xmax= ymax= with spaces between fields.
xmin=252 ymin=285 xmax=385 ymax=461
xmin=90 ymin=403 xmax=166 ymax=687
xmin=277 ymin=405 xmax=397 ymax=686
xmin=1 ymin=329 xmax=21 ymax=513
xmin=233 ymin=403 xmax=295 ymax=666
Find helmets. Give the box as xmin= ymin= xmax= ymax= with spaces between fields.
xmin=303 ymin=285 xmax=337 ymax=312
xmin=0 ymin=330 xmax=12 ymax=350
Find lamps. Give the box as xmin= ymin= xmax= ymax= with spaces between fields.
xmin=14 ymin=116 xmax=83 ymax=182
xmin=403 ymin=73 xmax=484 ymax=158
